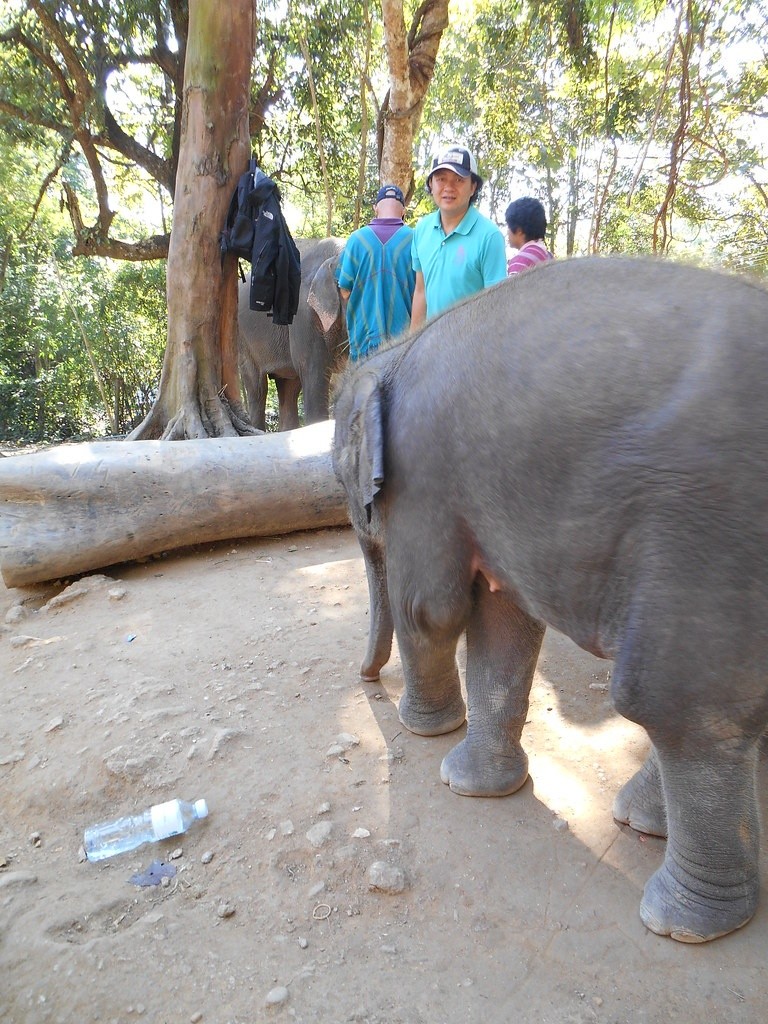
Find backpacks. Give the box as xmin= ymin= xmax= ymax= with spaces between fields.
xmin=219 ymin=158 xmax=257 ymax=263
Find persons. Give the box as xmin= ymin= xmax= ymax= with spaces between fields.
xmin=409 ymin=144 xmax=508 ymax=338
xmin=333 ymin=186 xmax=416 ymax=363
xmin=504 ymin=197 xmax=554 ymax=278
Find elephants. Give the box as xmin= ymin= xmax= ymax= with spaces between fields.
xmin=327 ymin=249 xmax=768 ymax=959
xmin=230 ymin=235 xmax=355 ymax=436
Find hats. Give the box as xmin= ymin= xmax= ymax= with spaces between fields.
xmin=425 ymin=144 xmax=477 ymax=193
xmin=375 ymin=185 xmax=405 ymax=221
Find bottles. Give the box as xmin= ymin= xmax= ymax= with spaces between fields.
xmin=83 ymin=798 xmax=208 ymax=863
